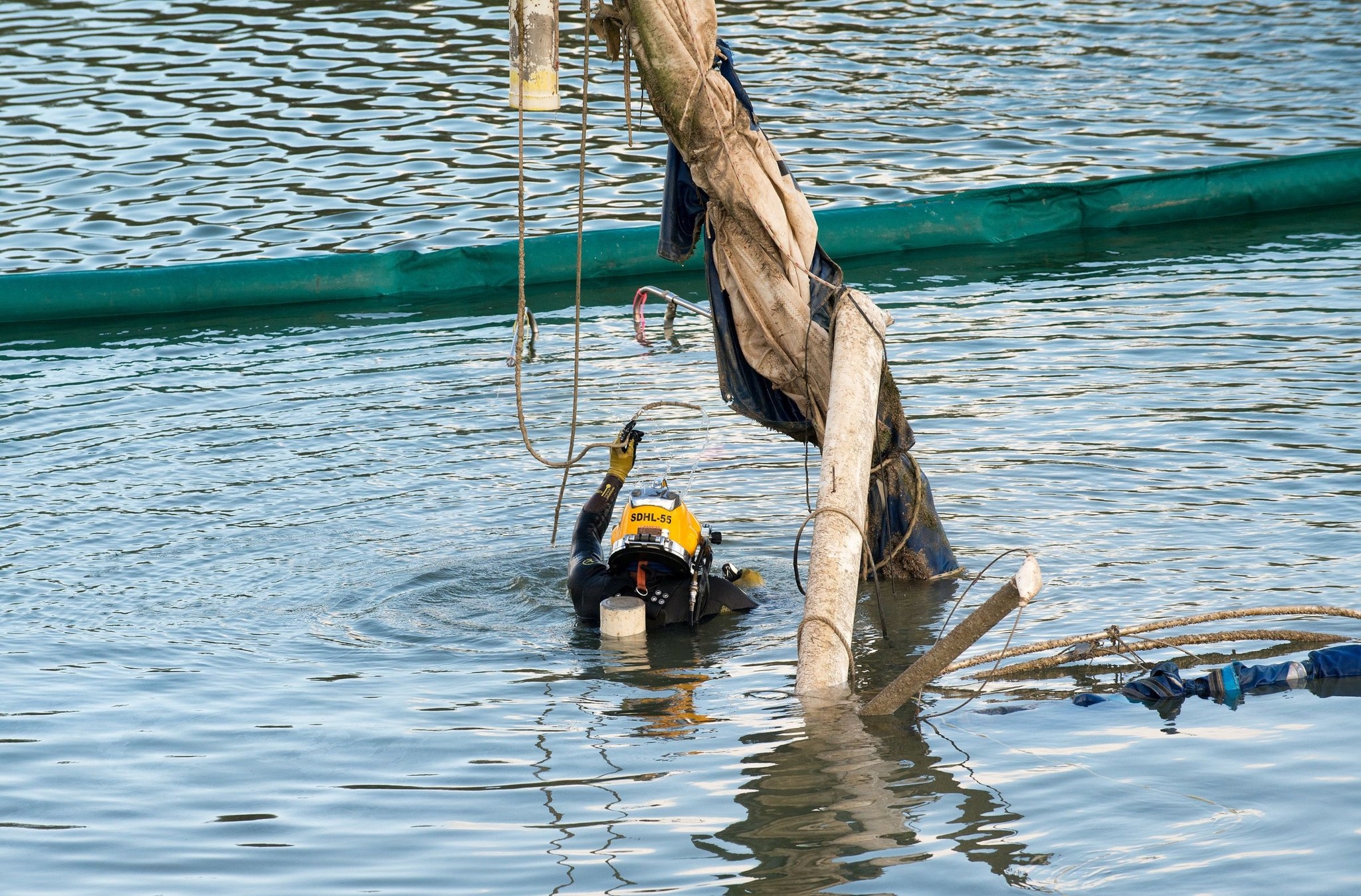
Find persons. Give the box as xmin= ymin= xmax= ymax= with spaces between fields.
xmin=567 ymin=419 xmax=763 ymax=636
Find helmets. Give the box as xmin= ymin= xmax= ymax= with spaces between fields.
xmin=607 ymin=476 xmax=702 ymax=573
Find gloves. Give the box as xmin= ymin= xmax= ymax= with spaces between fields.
xmin=606 ymin=420 xmax=644 ymax=482
xmin=732 ymin=568 xmax=765 ymax=589
